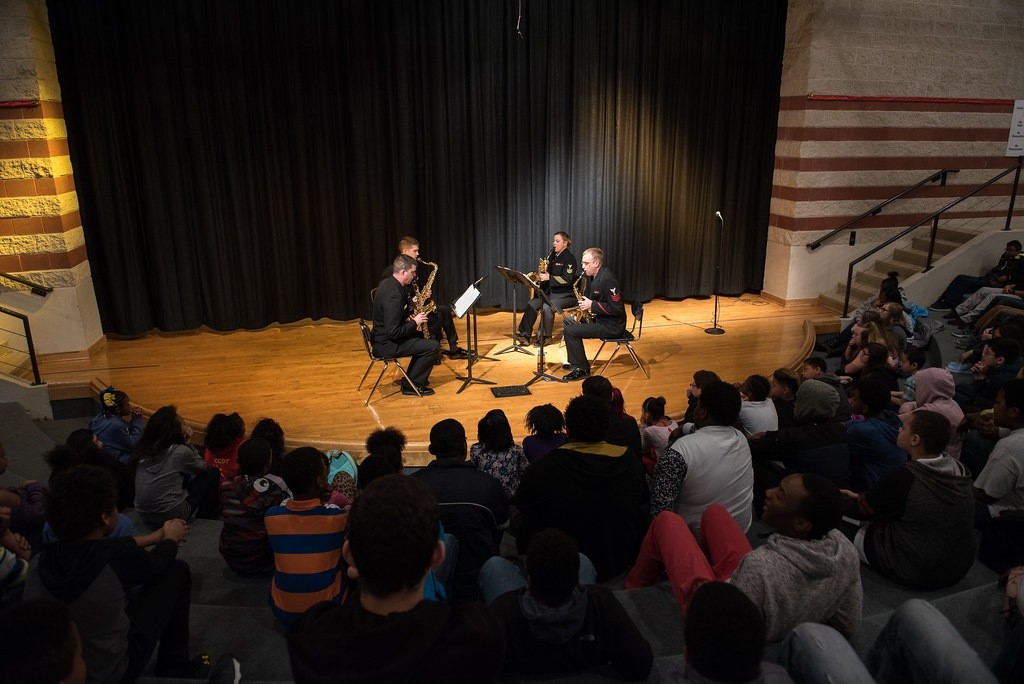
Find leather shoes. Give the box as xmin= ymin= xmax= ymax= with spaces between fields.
xmin=563 ymin=364 xmax=577 ymax=370
xmin=563 ymin=367 xmax=590 ymax=381
xmin=449 ymin=347 xmax=475 ymax=360
xmin=435 ymin=359 xmax=441 ymax=365
xmin=401 ymin=379 xmax=434 ymax=395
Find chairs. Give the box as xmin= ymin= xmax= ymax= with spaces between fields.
xmin=537 ymin=275 xmax=587 ymax=349
xmin=357 ymin=287 xmax=422 ymax=407
xmin=590 ymin=299 xmax=650 ymax=381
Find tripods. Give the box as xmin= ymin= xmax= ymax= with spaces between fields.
xmin=450 ymin=265 xmax=567 ymax=393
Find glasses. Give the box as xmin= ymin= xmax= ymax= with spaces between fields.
xmin=580 ymin=261 xmax=596 ymax=265
xmin=1004 ymin=248 xmax=1017 ymax=252
xmin=690 ymin=383 xmax=700 ymax=388
xmin=880 ymin=306 xmax=888 ymax=312
xmin=863 ymin=350 xmax=871 ymax=356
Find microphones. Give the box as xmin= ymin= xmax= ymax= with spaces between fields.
xmin=716 ymin=211 xmax=723 ymax=221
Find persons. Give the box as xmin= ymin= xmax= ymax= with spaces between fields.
xmin=516 ymin=231 xmax=578 ymax=346
xmin=399 ymin=236 xmax=475 ymax=360
xmin=0 ymin=240 xmax=1024 ymax=684
xmin=374 ymin=254 xmax=441 ymax=395
xmin=563 ymin=248 xmax=626 ymax=381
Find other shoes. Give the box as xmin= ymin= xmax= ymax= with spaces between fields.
xmin=952 ymin=326 xmax=975 ymax=338
xmin=957 ymin=339 xmax=977 ymax=350
xmin=929 ymin=298 xmax=951 ymax=312
xmin=943 ymin=311 xmax=959 ymax=319
xmin=515 ymin=332 xmax=530 ymax=345
xmin=534 ymin=335 xmax=552 ymax=346
xmin=948 ymin=318 xmax=965 ymax=325
xmin=209 ymin=652 xmax=242 ymax=684
xmin=180 ymin=652 xmax=210 ymax=679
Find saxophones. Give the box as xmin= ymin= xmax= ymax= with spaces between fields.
xmin=409 ymin=279 xmax=430 ymax=339
xmin=525 ymin=247 xmax=556 ymax=342
xmin=570 ymin=272 xmax=594 ymax=325
xmin=409 ymin=258 xmax=439 ymax=327
